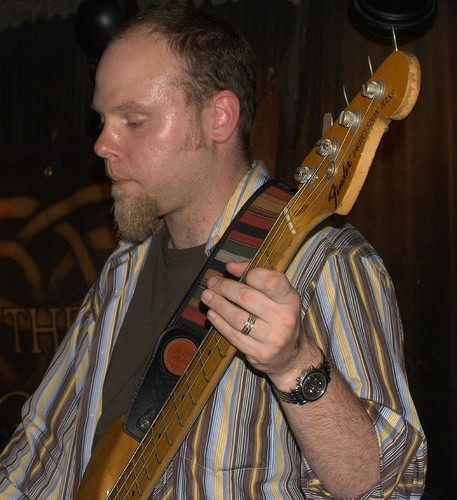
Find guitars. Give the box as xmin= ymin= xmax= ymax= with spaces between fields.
xmin=73 ymin=26 xmax=422 ymax=500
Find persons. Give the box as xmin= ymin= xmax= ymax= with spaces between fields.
xmin=0 ymin=1 xmax=429 ymax=500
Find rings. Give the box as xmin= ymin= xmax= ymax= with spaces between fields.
xmin=242 ymin=313 xmax=258 ymax=336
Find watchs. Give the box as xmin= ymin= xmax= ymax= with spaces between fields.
xmin=269 ymin=347 xmax=332 ymax=405
